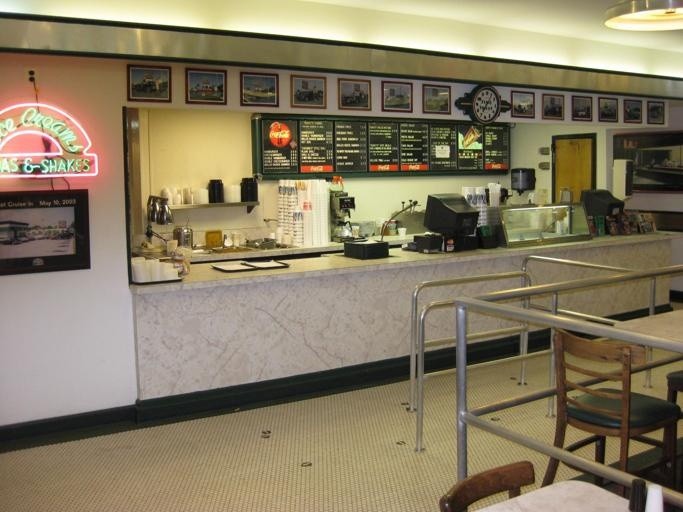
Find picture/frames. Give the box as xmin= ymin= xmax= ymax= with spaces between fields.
xmin=609 ymin=130 xmax=683 ymax=192
xmin=1 ymin=189 xmax=90 ymax=275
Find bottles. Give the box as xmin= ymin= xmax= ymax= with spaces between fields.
xmin=174 ymin=189 xmax=182 ymax=204
xmin=587 ymin=216 xmax=605 ymax=236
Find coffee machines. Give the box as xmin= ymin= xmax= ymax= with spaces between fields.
xmin=329 ymin=176 xmax=368 ymax=243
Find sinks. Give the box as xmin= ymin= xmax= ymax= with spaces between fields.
xmin=209 ymin=243 xmax=253 ymax=255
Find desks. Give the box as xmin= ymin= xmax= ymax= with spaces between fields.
xmin=612 ymin=310 xmax=683 ymax=355
xmin=469 ymin=479 xmax=648 ymax=512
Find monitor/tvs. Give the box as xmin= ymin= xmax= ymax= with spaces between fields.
xmin=580 ymin=189 xmax=625 ymax=218
xmin=422 ymin=192 xmax=479 ymax=252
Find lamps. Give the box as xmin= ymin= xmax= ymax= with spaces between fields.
xmin=602 ymin=0 xmax=683 ymax=32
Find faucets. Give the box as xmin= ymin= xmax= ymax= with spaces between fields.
xmin=223 ymin=233 xmax=228 ymax=247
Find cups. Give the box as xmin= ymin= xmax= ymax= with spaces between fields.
xmin=351 ymin=225 xmax=359 ymax=237
xmin=461 ymin=186 xmax=487 ymax=236
xmin=397 ymin=228 xmax=407 ymax=237
xmin=462 ymin=126 xmax=480 ymax=148
xmin=233 ymin=233 xmax=241 ymax=246
xmin=183 ymin=177 xmax=258 ymax=205
xmin=489 ymin=183 xmax=502 ymax=206
xmin=270 ymin=179 xmax=331 ymax=246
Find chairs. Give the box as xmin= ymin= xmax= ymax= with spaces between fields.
xmin=667 ymin=371 xmax=683 ymax=486
xmin=541 ymin=328 xmax=683 ymax=498
xmin=439 ymin=462 xmax=535 ymax=507
